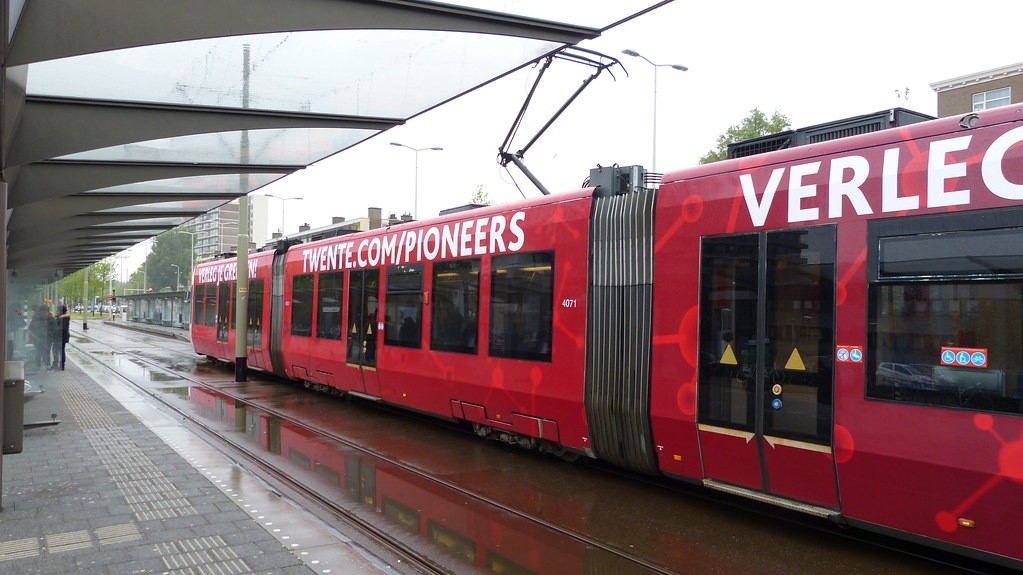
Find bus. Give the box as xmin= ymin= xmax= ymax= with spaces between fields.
xmin=185 ymin=42 xmax=1022 ymax=574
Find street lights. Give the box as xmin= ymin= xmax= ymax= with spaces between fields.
xmin=621 ymin=48 xmax=689 ymax=174
xmin=206 ymin=220 xmax=240 ymax=254
xmin=178 ymin=230 xmax=208 ymax=274
xmin=389 ymin=141 xmax=444 ymax=220
xmin=264 ymin=193 xmax=304 ymax=238
xmin=170 ymin=262 xmax=180 ymax=293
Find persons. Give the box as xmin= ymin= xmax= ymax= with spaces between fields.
xmin=364 ymin=315 xmax=378 ymax=360
xmin=27 ymin=303 xmax=71 ymax=371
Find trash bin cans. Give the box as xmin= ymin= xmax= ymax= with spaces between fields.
xmin=3 ymin=361 xmax=25 ymax=454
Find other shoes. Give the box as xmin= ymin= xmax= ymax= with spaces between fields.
xmin=57 ymin=363 xmax=65 ymax=370
xmin=49 ymin=362 xmax=58 ymax=370
